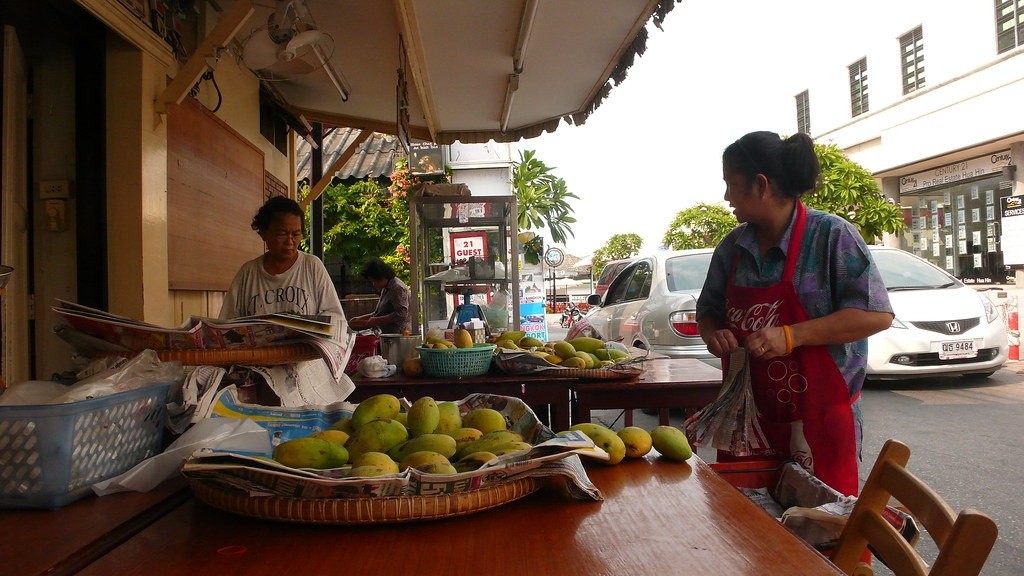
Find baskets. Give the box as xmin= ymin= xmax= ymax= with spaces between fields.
xmin=1 ymin=383 xmax=169 ymax=510
xmin=416 ymin=345 xmax=498 ymax=378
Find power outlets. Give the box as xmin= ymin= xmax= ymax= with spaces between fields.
xmin=39 ymin=180 xmax=72 ymax=199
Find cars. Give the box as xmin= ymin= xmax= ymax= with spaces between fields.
xmin=520 ymin=315 xmax=544 ymax=324
xmin=587 ymin=248 xmax=721 ymax=358
xmin=546 ymin=302 xmax=593 ymax=315
xmin=864 ymin=244 xmax=1010 ymax=382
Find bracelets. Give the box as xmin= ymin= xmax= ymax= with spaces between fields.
xmin=782 ymin=324 xmax=792 ymax=354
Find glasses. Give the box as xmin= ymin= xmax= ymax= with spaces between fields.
xmin=271 ymin=234 xmax=304 ymax=243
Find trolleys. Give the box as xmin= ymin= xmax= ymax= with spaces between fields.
xmin=564 ymin=321 xmax=624 ymax=343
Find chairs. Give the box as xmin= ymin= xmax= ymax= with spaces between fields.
xmin=827 ymin=438 xmax=999 ymax=575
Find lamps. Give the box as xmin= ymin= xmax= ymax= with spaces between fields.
xmin=311 ymin=44 xmax=351 ymax=102
xmin=500 ymin=74 xmax=520 ymax=134
xmin=512 ymin=1 xmax=540 ymax=73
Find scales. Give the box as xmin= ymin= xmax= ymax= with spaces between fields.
xmin=444 ymin=285 xmax=494 ymax=339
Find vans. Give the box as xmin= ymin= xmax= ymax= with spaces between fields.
xmin=596 ymin=259 xmax=635 ymax=298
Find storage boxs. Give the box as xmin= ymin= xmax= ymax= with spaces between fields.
xmin=704 ymin=461 xmax=920 ymax=564
xmin=0 ymin=379 xmax=180 ymax=512
xmin=343 ymin=335 xmax=378 ymax=376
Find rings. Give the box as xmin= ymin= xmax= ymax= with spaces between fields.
xmin=759 ymin=346 xmax=766 ymax=352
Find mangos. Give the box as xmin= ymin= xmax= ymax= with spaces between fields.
xmin=251 ymin=395 xmax=532 ymax=474
xmin=423 ymin=329 xmax=473 ymax=349
xmin=556 ymin=423 xmax=692 ymax=464
xmin=403 ymin=359 xmax=422 ymax=376
xmin=494 ymin=331 xmax=628 ymax=369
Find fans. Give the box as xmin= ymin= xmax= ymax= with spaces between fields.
xmin=214 ymin=0 xmax=337 ymax=81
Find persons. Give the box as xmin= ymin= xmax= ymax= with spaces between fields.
xmin=347 ymin=258 xmax=412 ymax=356
xmin=695 ymin=131 xmax=895 ymax=565
xmin=217 ymin=196 xmax=345 ymax=329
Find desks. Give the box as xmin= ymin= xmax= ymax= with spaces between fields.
xmin=348 ymin=348 xmax=723 ymax=436
xmin=66 ymin=452 xmax=849 ymax=576
xmin=0 ymin=461 xmax=190 ymax=576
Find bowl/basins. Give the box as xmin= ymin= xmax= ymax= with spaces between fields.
xmin=428 ymin=265 xmax=449 ymax=275
xmin=380 ymin=334 xmax=421 ymax=368
